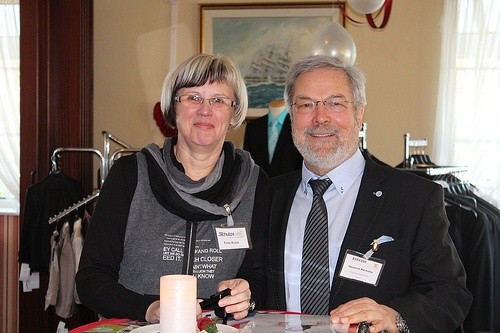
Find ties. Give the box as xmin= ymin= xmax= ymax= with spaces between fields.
xmin=300 ymin=177 xmax=333 ymax=316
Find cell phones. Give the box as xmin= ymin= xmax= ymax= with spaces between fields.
xmin=200 ymin=288 xmax=231 ymax=308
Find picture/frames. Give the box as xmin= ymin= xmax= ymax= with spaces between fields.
xmin=196 ymin=0 xmax=346 ymax=126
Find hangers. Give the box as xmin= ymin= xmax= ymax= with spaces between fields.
xmin=26 ymin=138 xmax=500 ymax=253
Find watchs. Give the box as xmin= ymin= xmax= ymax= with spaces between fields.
xmin=396 ymin=313 xmax=410 ymax=333
xmin=250 ymin=299 xmax=257 ymax=312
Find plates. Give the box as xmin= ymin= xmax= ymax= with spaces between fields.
xmin=129 ymin=323 xmax=241 ymax=333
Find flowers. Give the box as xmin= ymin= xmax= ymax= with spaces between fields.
xmin=197 ymin=317 xmax=218 ymax=333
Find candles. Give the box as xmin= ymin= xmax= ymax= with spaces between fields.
xmin=159 ymin=274 xmax=197 ymax=333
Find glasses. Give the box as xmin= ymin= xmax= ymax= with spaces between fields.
xmin=173 ymin=94 xmax=238 ymax=109
xmin=290 ymin=97 xmax=357 ymax=114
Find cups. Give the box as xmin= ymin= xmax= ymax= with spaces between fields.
xmin=159 ymin=274 xmax=196 ymax=333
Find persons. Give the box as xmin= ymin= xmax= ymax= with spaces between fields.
xmin=243 ymin=100 xmax=302 ymax=178
xmin=75 ymin=55 xmax=268 ymax=323
xmin=236 ymin=58 xmax=473 ymax=333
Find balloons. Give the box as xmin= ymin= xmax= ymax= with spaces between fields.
xmin=306 ymin=22 xmax=357 ymax=66
xmin=349 ymin=0 xmax=386 ymax=14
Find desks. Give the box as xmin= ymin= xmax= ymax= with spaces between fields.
xmin=65 ymin=309 xmax=391 ymax=333
